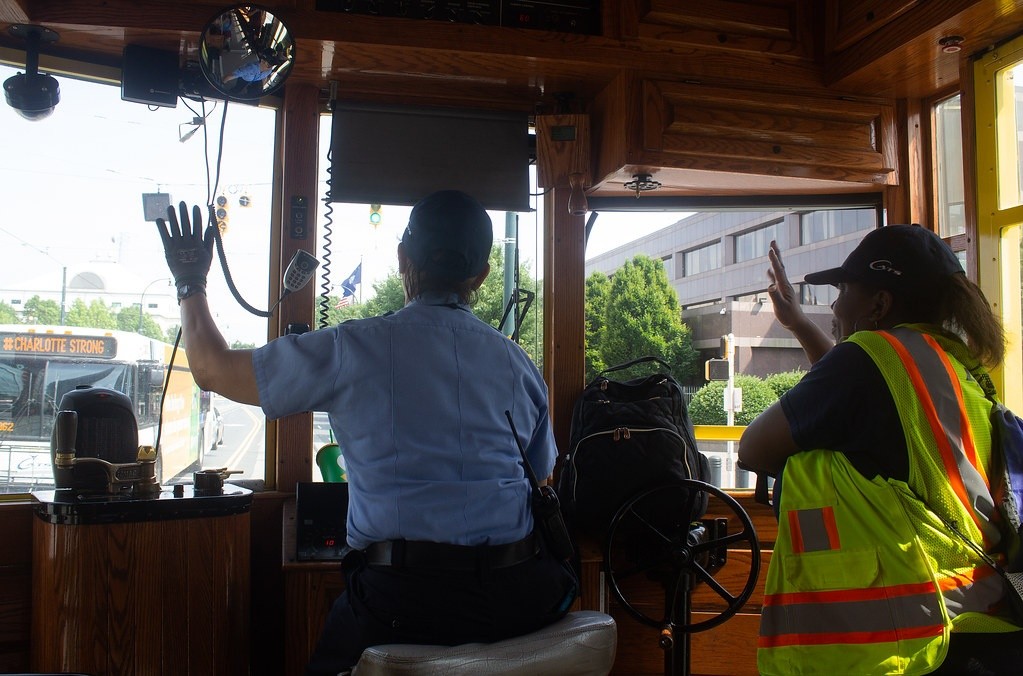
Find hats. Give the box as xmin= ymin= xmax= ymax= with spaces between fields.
xmin=404 ymin=189 xmax=493 ymax=273
xmin=804 ymin=225 xmax=967 ymax=304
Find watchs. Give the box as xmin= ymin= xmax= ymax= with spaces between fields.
xmin=177 ymin=285 xmax=206 ymax=301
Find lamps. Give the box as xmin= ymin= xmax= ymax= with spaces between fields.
xmin=759 ymin=298 xmax=767 ymax=304
xmin=3 ymin=24 xmax=62 ymax=121
xmin=720 ymin=308 xmax=726 ymax=314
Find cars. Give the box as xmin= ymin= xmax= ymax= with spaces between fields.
xmin=213 ymin=407 xmax=224 ymax=450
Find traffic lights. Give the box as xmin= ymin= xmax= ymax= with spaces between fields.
xmin=215 ymin=195 xmax=229 ymax=236
xmin=238 ymin=196 xmax=250 ymax=207
xmin=719 ymin=335 xmax=728 ymax=358
xmin=704 ymin=359 xmax=730 ymax=381
xmin=369 ymin=204 xmax=383 ymax=226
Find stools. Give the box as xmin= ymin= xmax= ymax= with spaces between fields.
xmin=350 ymin=611 xmax=618 ymax=676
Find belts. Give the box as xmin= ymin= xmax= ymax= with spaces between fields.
xmin=367 ymin=532 xmax=538 ymax=572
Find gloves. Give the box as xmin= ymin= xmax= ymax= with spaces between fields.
xmin=155 ymin=201 xmax=217 ymax=302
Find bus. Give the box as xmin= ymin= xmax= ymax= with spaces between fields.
xmin=0 ymin=322 xmax=217 ymax=502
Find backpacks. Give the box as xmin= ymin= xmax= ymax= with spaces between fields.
xmin=906 ymin=365 xmax=1022 ymax=599
xmin=552 ymin=356 xmax=713 ymax=549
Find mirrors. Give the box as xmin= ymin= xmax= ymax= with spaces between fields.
xmin=198 ymin=7 xmax=296 ymax=98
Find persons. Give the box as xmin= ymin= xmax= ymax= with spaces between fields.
xmin=735 ymin=220 xmax=1013 ymax=676
xmin=155 ymin=188 xmax=581 ymax=676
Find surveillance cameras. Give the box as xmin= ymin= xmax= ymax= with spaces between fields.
xmin=4 ymin=74 xmax=61 ymax=121
